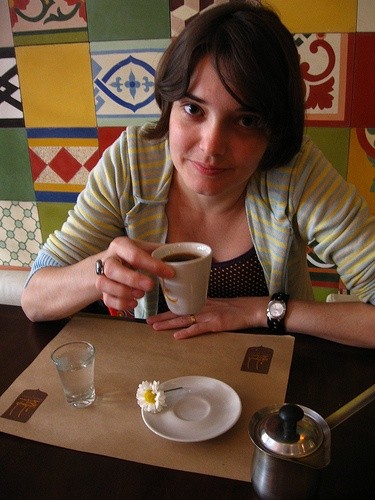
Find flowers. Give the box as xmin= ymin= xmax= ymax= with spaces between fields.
xmin=134 ymin=379 xmax=185 ymax=414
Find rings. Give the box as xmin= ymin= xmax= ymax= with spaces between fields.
xmin=190 ymin=314 xmax=195 ymax=324
xmin=95 ymin=256 xmax=120 ymax=275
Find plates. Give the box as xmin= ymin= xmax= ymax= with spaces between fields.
xmin=140 ymin=374 xmax=242 ymax=442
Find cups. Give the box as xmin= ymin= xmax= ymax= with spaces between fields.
xmin=152 ymin=242 xmax=212 ymax=316
xmin=50 ymin=341 xmax=94 ymax=407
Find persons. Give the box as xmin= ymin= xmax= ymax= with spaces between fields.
xmin=22 ymin=2 xmax=375 ymax=348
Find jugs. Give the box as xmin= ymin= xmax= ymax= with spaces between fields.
xmin=248 ymin=401 xmax=332 ymax=500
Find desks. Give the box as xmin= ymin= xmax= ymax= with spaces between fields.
xmin=0 ymin=302 xmax=375 ymax=500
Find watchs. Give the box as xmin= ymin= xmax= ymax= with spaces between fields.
xmin=266 ymin=291 xmax=290 ymax=330
